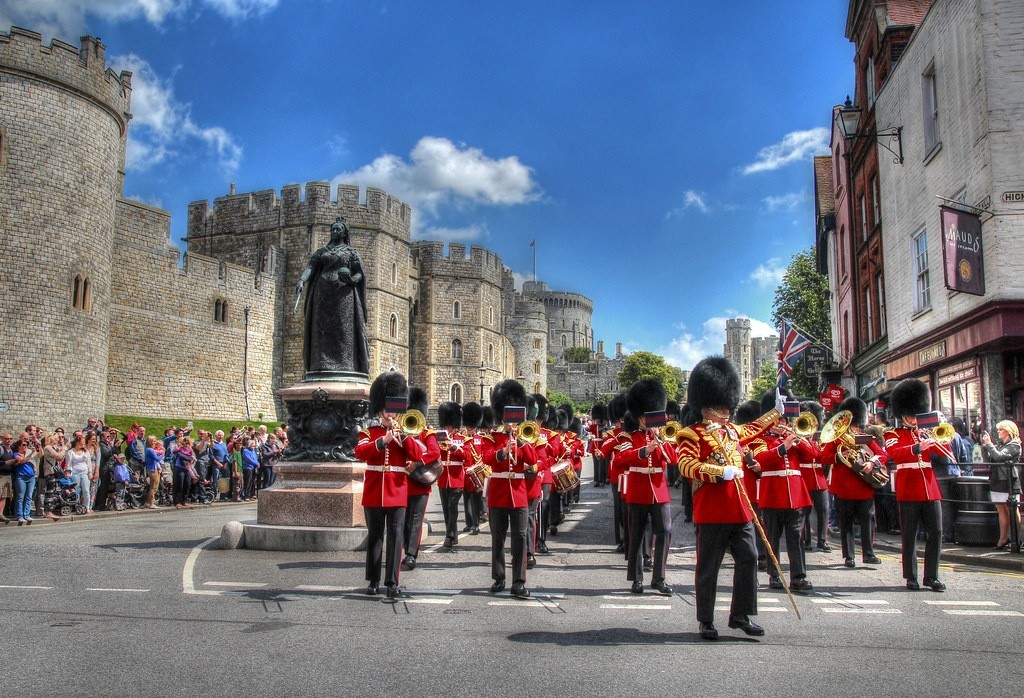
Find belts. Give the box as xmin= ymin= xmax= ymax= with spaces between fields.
xmin=735 ymin=470 xmax=745 ymax=478
xmin=618 ymin=467 xmax=664 ymax=494
xmin=491 ymin=472 xmax=525 ymax=479
xmin=761 ymin=470 xmax=802 ymax=476
xmin=897 ymin=462 xmax=932 ymax=469
xmin=366 ymin=460 xmax=466 ymax=475
xmin=800 ymin=463 xmax=822 ymax=468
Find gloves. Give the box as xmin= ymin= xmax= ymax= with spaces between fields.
xmin=722 ymin=466 xmax=738 ymax=480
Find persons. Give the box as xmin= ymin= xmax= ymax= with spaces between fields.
xmin=979 ymin=420 xmax=1022 ymax=550
xmin=298 ymin=220 xmax=370 ymax=374
xmin=0 ymin=417 xmax=288 ymax=523
xmin=676 ymin=405 xmax=766 ymax=641
xmin=354 ymin=405 xmax=951 ymax=599
xmin=925 ymin=411 xmax=974 ymax=475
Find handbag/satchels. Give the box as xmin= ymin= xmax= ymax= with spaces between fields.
xmin=53 ymin=463 xmax=64 ymax=476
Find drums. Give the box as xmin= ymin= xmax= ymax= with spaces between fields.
xmin=550 ymin=458 xmax=580 ymax=494
xmin=465 ymin=460 xmax=492 ymax=492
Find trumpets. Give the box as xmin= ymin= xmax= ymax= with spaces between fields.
xmin=438 ymin=430 xmax=454 ymax=451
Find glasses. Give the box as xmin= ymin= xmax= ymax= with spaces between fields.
xmin=89 ymin=420 xmax=96 ymax=423
xmin=110 ymin=433 xmax=116 ymax=435
xmin=5 ymin=438 xmax=13 ymax=441
xmin=105 ymin=436 xmax=111 ymax=438
xmin=199 ymin=433 xmax=205 ymax=435
xmin=23 ymin=443 xmax=29 ymax=447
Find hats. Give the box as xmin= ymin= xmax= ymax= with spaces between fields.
xmin=366 ymin=354 xmax=933 ymax=444
xmin=117 ymin=453 xmax=125 ymax=458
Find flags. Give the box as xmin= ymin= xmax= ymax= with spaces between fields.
xmin=529 ymin=240 xmax=535 ymax=248
xmin=778 ymin=319 xmax=809 ymax=386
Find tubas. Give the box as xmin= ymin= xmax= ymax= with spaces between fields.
xmin=820 ymin=410 xmax=890 ymax=489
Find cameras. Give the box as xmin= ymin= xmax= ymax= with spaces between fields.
xmin=983 ymin=431 xmax=987 ymax=435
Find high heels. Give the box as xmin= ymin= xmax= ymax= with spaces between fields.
xmin=993 ymin=538 xmax=1012 ymax=550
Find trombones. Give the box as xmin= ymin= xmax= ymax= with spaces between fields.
xmin=649 ymin=420 xmax=682 ymax=463
xmin=777 ymin=411 xmax=818 ymax=449
xmin=912 ymin=420 xmax=957 ymax=465
xmin=507 ymin=420 xmax=540 ymax=464
xmin=388 ymin=409 xmax=427 ymax=447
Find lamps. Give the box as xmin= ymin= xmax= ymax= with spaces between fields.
xmin=834 ymin=95 xmax=904 ymax=165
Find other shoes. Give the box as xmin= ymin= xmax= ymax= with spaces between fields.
xmin=0 ymin=494 xmax=260 ymax=524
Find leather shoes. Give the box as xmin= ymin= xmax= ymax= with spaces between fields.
xmin=699 ymin=620 xmax=718 ymax=639
xmin=363 ymin=494 xmax=959 ymax=600
xmin=727 ymin=615 xmax=764 ymax=637
